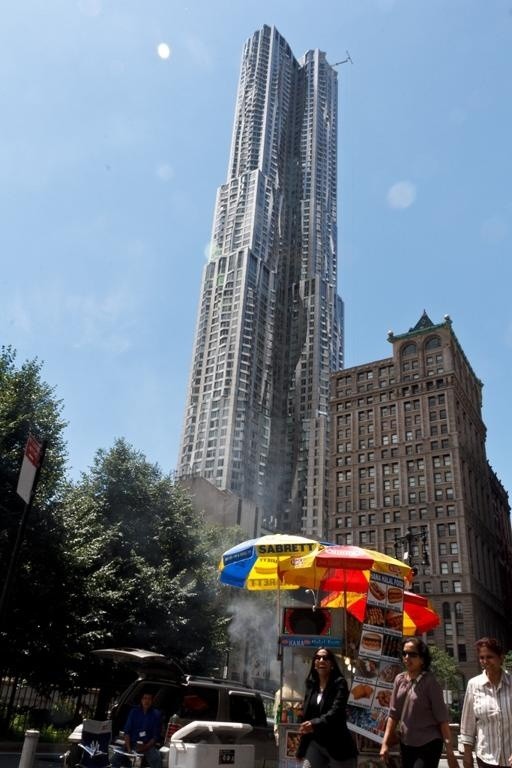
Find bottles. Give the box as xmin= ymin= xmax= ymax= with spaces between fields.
xmin=164 ymin=714 xmax=181 ymax=746
xmin=383 ymin=635 xmax=402 ymax=657
xmin=276 ymin=704 xmax=304 ymax=724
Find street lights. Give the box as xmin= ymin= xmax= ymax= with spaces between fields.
xmin=394 ymin=524 xmax=431 ymax=594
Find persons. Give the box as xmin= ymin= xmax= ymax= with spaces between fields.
xmin=460 ymin=639 xmax=512 ymax=768
xmin=111 ymin=693 xmax=162 ymax=768
xmin=380 ymin=638 xmax=460 ymax=768
xmin=296 ymin=648 xmax=360 ymax=768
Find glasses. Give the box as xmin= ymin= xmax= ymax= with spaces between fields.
xmin=314 ymin=654 xmax=332 ymax=661
xmin=402 ymin=651 xmax=419 ymax=658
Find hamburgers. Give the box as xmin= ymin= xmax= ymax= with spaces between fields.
xmin=385 ymin=611 xmax=403 ymax=629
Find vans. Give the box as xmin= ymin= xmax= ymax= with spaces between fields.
xmin=90 ymin=645 xmax=279 ymax=767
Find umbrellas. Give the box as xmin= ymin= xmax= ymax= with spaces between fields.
xmin=218 ymin=532 xmax=441 ymax=637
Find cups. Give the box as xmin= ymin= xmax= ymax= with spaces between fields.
xmin=137 ymin=740 xmax=144 ymax=753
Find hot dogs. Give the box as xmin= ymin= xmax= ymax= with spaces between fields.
xmin=369 ymin=580 xmax=385 ymax=601
xmin=388 ymin=588 xmax=402 ymax=602
xmin=362 ymin=633 xmax=381 ymax=650
